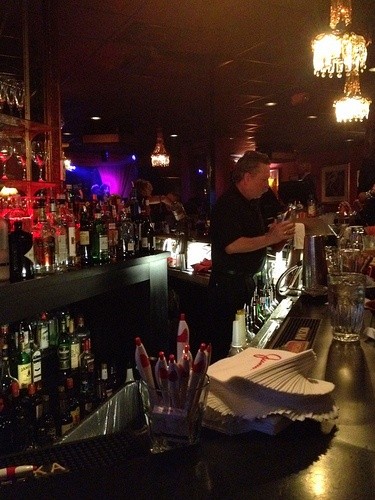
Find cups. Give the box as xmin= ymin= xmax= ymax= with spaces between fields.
xmin=325 ymin=272 xmax=365 ymax=341
xmin=138 ymin=374 xmax=211 ymax=452
xmin=324 ymin=223 xmax=375 ymax=275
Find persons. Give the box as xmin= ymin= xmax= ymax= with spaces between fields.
xmin=207 ymin=152 xmax=296 ymax=358
xmin=138 ymin=181 xmax=177 ymax=222
xmin=357 ymin=170 xmax=375 ymax=227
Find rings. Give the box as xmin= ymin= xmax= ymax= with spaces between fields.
xmin=283 ymin=231 xmax=286 ymax=234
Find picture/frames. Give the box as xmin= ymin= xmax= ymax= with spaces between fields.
xmin=322 ymin=163 xmax=351 ymax=204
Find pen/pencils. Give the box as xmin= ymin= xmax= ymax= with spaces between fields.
xmin=134 ymin=315 xmax=212 ymax=410
xmin=0 ymin=465 xmax=38 ymax=477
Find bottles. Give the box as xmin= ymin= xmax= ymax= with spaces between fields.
xmin=226 ymin=253 xmax=279 ymax=358
xmin=307 ymin=194 xmax=317 ymax=217
xmin=1 ymin=185 xmax=212 ymax=283
xmin=0 ymin=311 xmax=136 ymax=457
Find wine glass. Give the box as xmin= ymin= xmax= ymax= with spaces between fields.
xmin=0 ymin=72 xmax=26 ymax=120
xmin=0 ymin=131 xmax=47 ymax=182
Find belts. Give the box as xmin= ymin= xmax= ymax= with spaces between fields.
xmin=212 ymin=267 xmax=252 ymax=276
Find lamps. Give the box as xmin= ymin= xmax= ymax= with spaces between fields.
xmin=150 ymin=128 xmax=171 ymax=167
xmin=306 ymin=0 xmax=374 ymax=126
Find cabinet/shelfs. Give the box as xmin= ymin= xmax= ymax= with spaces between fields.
xmin=0 ymin=0 xmax=64 ymax=265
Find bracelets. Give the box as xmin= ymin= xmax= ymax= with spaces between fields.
xmin=365 ymin=190 xmax=373 ymax=200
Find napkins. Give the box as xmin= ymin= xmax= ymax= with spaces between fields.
xmin=202 ymin=346 xmax=341 ymax=436
